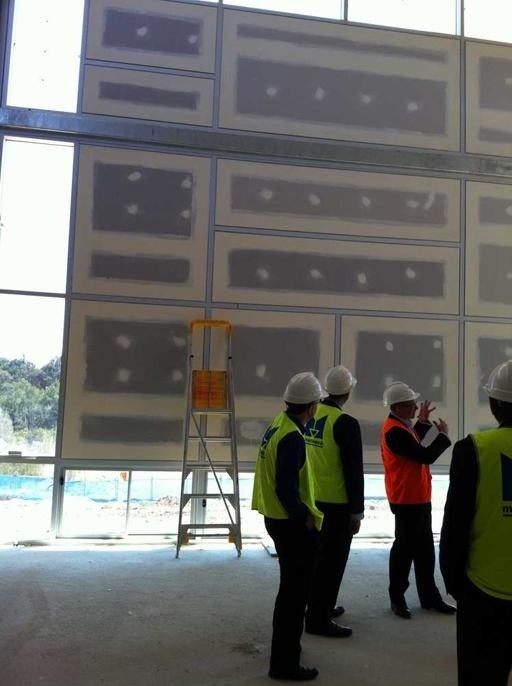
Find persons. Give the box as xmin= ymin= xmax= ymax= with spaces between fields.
xmin=439 ymin=359 xmax=512 ymax=686
xmin=251 ymin=372 xmax=325 ymax=681
xmin=380 ymin=382 xmax=457 ymax=619
xmin=304 ymin=366 xmax=364 ymax=638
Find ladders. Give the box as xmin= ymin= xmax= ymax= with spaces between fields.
xmin=174 ymin=319 xmax=244 ymax=560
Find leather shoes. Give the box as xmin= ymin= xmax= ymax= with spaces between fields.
xmin=268 ymin=665 xmax=320 ymax=682
xmin=390 ymin=601 xmax=412 ymax=619
xmin=305 ymin=620 xmax=354 ymax=639
xmin=423 ymin=599 xmax=457 ymax=615
xmin=331 ymin=604 xmax=346 ymax=618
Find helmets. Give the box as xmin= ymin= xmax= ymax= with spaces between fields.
xmin=382 ymin=380 xmax=421 ymax=408
xmin=324 ymin=365 xmax=358 ymax=396
xmin=481 ymin=359 xmax=511 ymax=404
xmin=283 ymin=370 xmax=330 ymax=407
